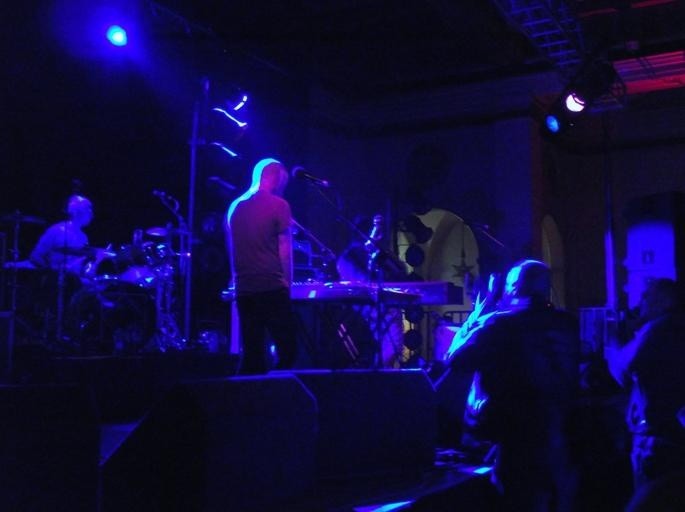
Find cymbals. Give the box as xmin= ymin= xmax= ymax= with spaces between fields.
xmin=147 ymin=227 xmax=192 ymax=238
xmin=52 ymin=246 xmax=115 ymax=257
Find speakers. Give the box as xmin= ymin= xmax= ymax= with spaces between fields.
xmin=180 ymin=373 xmax=318 ymax=506
xmin=0 ymin=355 xmax=208 ymax=511
xmin=271 ymin=369 xmax=436 ymax=480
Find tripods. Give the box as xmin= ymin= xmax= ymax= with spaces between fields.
xmin=147 ymin=267 xmax=185 ymax=354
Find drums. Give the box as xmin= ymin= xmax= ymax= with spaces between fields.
xmin=72 ymin=278 xmax=157 ymax=353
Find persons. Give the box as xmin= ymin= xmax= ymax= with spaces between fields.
xmin=26 ymin=194 xmax=96 ymax=332
xmin=224 ymin=156 xmax=294 ymax=372
xmin=601 ymin=274 xmax=684 ymax=511
xmin=443 ymin=258 xmax=583 ymax=511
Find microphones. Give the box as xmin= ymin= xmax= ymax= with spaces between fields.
xmin=366 ymin=214 xmax=383 ymax=247
xmin=292 ymin=166 xmax=330 ymax=187
